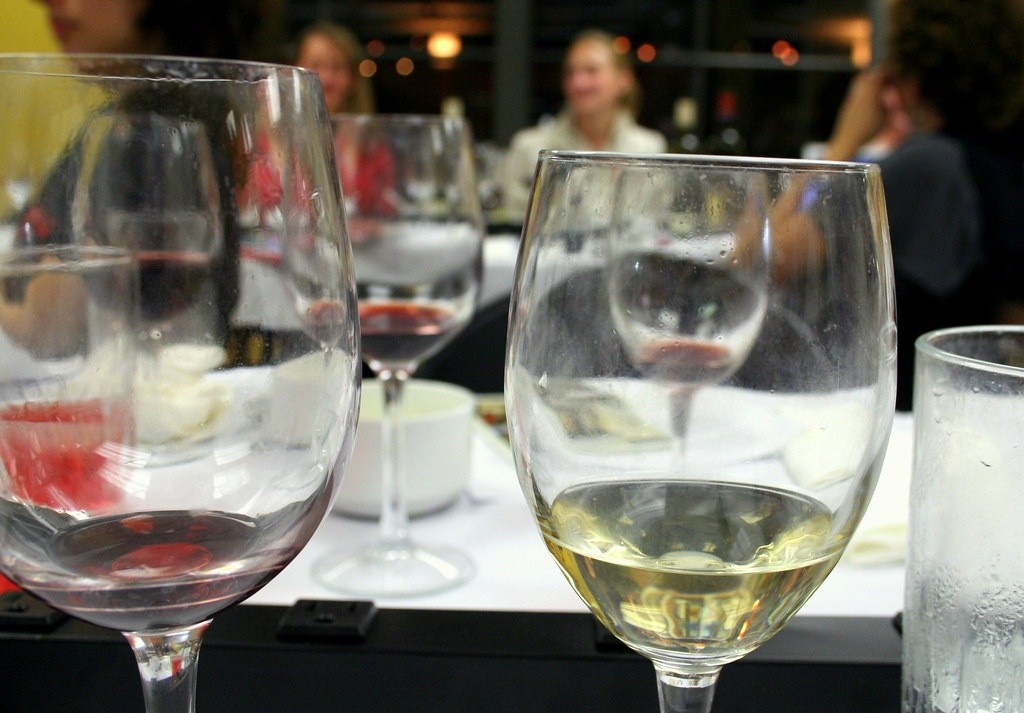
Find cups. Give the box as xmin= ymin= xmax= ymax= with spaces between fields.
xmin=900 ymin=324 xmax=1024 ymax=713
xmin=1 ymin=245 xmax=139 ymax=598
xmin=327 ymin=375 xmax=478 ymax=519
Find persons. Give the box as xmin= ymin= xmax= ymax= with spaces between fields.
xmin=1 ymin=0 xmax=1024 ymax=419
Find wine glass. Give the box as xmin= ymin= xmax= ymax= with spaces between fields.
xmin=277 ymin=111 xmax=485 ymax=600
xmin=501 ymin=147 xmax=896 ymax=713
xmin=2 ymin=52 xmax=365 ymax=713
